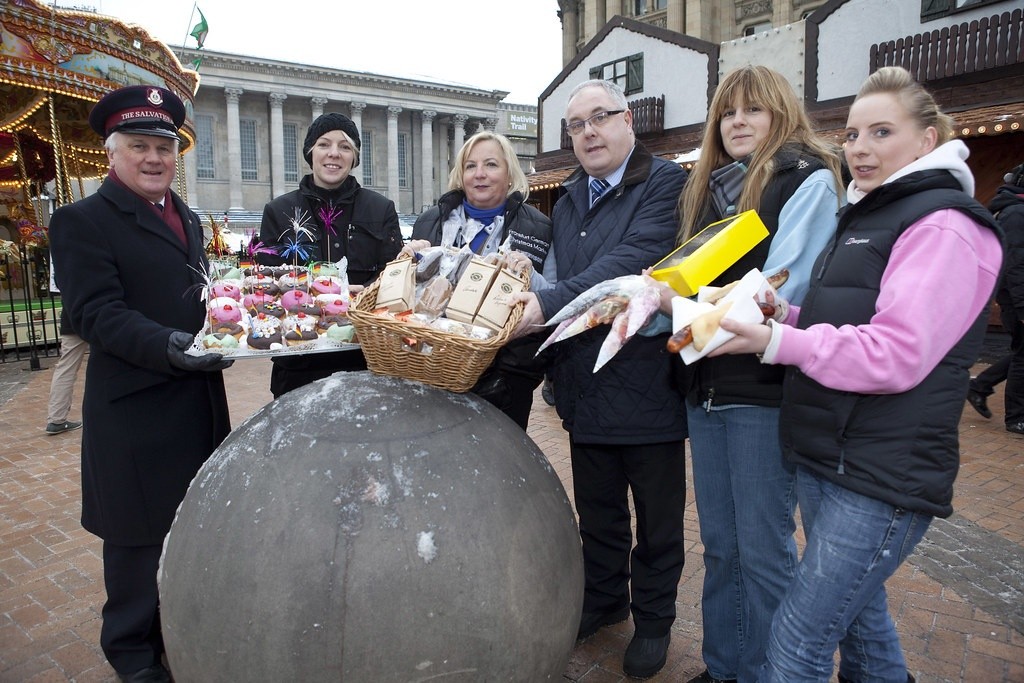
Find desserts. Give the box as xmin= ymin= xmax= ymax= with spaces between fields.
xmin=200 ymin=261 xmax=354 ymax=347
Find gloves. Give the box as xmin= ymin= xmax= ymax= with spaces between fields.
xmin=167 ymin=331 xmax=234 ymax=372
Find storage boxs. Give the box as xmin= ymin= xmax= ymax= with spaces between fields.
xmin=648 ymin=209 xmax=770 ymax=299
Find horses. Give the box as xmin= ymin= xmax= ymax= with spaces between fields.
xmin=4 ymin=198 xmax=49 ymax=248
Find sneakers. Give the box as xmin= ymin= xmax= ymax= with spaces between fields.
xmin=578 ymin=604 xmax=631 ymax=639
xmin=623 ymin=633 xmax=671 ymax=679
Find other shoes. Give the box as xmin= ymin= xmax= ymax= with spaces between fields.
xmin=967 ymin=380 xmax=992 ymax=418
xmin=46 ymin=420 xmax=82 ymax=433
xmin=686 ymin=669 xmax=738 ymax=683
xmin=1006 ymin=421 xmax=1024 ymax=434
xmin=123 ymin=664 xmax=171 ymax=683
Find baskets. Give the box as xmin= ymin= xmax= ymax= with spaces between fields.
xmin=347 ymin=246 xmax=529 ymax=393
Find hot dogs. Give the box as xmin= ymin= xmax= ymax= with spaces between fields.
xmin=668 ymin=269 xmax=790 ymax=353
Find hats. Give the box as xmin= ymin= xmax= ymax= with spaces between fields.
xmin=304 ymin=113 xmax=361 ymax=168
xmin=89 ymin=85 xmax=186 ymax=142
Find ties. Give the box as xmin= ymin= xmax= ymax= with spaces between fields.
xmin=590 ymin=178 xmax=610 ymax=206
xmin=154 ymin=204 xmax=164 ymax=211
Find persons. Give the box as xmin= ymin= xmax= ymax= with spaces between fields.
xmin=259 ymin=112 xmax=403 ymax=402
xmin=510 ymin=78 xmax=688 ymax=680
xmin=46 ymin=271 xmax=91 ymax=434
xmin=641 ymin=63 xmax=846 ymax=683
xmin=967 ymin=163 xmax=1024 ymax=435
xmin=405 ymin=129 xmax=557 ymax=433
xmin=707 ymin=66 xmax=1007 ymax=683
xmin=47 ymin=85 xmax=236 ymax=683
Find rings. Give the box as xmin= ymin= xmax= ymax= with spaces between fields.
xmin=524 ymin=332 xmax=529 ymax=336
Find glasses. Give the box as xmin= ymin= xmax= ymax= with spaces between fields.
xmin=566 ymin=110 xmax=626 ymax=137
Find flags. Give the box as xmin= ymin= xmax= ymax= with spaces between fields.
xmin=184 ymin=57 xmax=202 ymax=71
xmin=191 ymin=6 xmax=209 ymax=50
